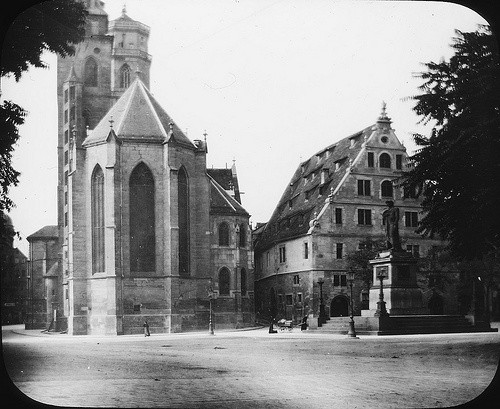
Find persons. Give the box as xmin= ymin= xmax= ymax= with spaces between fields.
xmin=269 ymin=317 xmax=276 ymax=333
xmin=301 ymin=314 xmax=309 ymax=331
xmin=143 ymin=320 xmax=151 ymax=337
xmin=382 ymin=202 xmax=404 ymax=252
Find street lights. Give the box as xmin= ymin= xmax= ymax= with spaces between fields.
xmin=373 ymin=267 xmax=389 ymax=316
xmin=344 ymin=266 xmax=357 ymax=337
xmin=316 ymin=278 xmax=325 ymax=322
